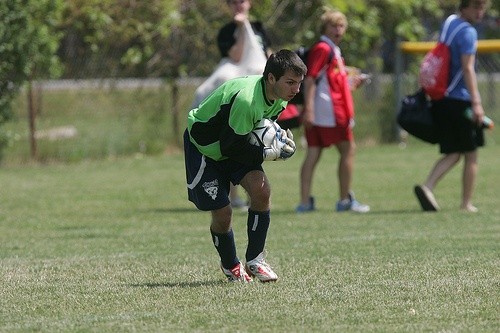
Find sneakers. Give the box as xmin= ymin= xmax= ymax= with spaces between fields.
xmin=220 ymin=261 xmax=253 ymax=284
xmin=245 ymin=252 xmax=278 ymax=282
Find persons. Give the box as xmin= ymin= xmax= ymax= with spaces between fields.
xmin=396 ymin=0 xmax=495 ymax=212
xmin=226 ymin=0 xmax=267 ymax=77
xmin=192 ymin=20 xmax=251 ymax=211
xmin=296 ymin=9 xmax=371 ymax=214
xmin=183 ymin=48 xmax=307 ymax=284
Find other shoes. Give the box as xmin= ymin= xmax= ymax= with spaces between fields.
xmin=414 ymin=184 xmax=440 ymax=212
xmin=296 ymin=198 xmax=314 ymax=212
xmin=337 ymin=193 xmax=371 ymax=213
xmin=230 ymin=201 xmax=249 ymax=211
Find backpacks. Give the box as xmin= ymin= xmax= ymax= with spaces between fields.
xmin=421 ymin=16 xmax=476 ymax=99
xmin=290 ymin=43 xmax=334 ymax=104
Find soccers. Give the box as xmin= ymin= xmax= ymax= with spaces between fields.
xmin=247 ymin=118 xmax=281 ymax=148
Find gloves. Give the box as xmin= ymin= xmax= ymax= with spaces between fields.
xmin=262 ymin=129 xmax=295 ymax=160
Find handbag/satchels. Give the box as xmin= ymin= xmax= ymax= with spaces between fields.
xmin=396 ymin=92 xmax=440 ymax=144
